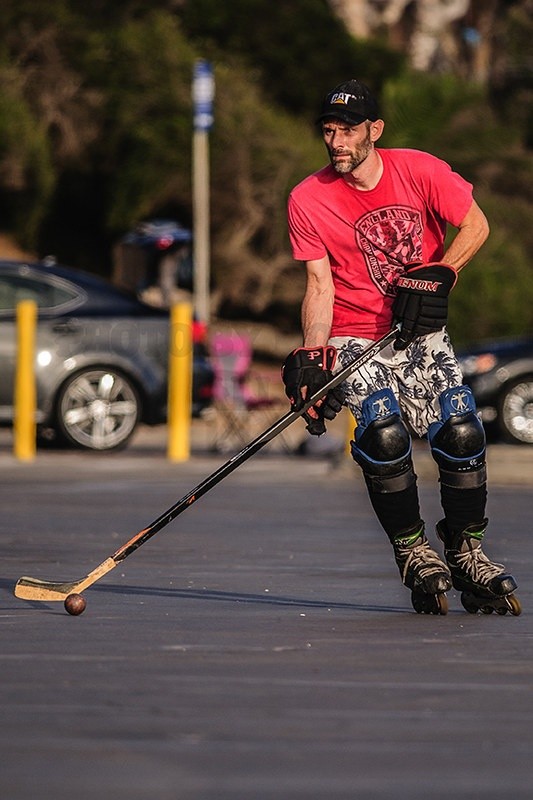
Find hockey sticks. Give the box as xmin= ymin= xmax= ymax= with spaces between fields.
xmin=14 ymin=321 xmax=402 ymax=601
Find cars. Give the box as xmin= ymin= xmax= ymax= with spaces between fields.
xmin=0 ymin=258 xmax=219 ymax=455
xmin=450 ymin=329 xmax=533 ymax=446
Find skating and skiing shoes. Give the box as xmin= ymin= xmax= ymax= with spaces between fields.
xmin=390 ymin=518 xmax=454 ymax=617
xmin=435 ymin=517 xmax=522 ymax=615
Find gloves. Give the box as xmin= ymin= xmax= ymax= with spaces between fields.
xmin=389 ymin=260 xmax=458 ymax=351
xmin=281 ymin=344 xmax=347 ymax=438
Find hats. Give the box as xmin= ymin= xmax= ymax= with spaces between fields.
xmin=315 ymin=77 xmax=380 ymax=125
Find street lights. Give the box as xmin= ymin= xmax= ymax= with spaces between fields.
xmin=190 ymin=60 xmax=216 ymax=325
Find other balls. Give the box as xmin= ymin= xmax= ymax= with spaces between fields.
xmin=64 ymin=593 xmax=86 ymax=616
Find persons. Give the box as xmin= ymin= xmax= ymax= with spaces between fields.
xmin=205 ymin=309 xmax=254 ymax=457
xmin=283 ymin=76 xmax=522 ymax=619
